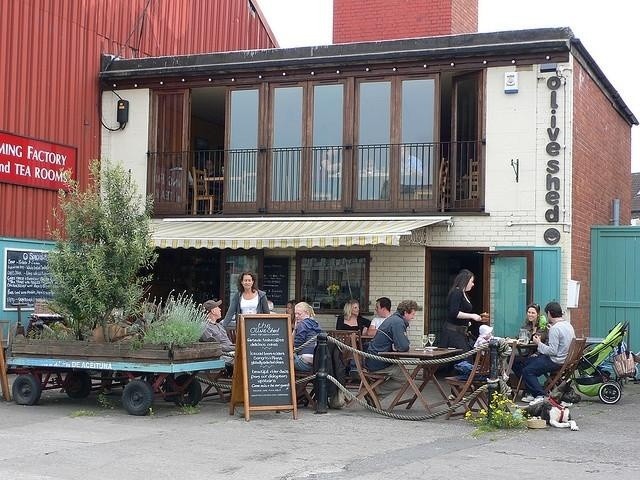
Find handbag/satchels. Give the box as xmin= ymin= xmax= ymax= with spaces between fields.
xmin=613 ymin=351 xmax=635 ymax=378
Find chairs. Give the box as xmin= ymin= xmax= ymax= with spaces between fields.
xmin=414 ymin=157 xmax=479 ymax=199
xmin=202 ymin=368 xmax=232 ymax=401
xmin=191 ymin=166 xmax=222 ymax=215
xmin=445 ymin=337 xmax=587 ymax=420
xmin=294 ymin=330 xmax=386 ymax=410
xmin=225 ymin=327 xmax=236 ymax=344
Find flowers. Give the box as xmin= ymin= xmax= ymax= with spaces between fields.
xmin=327 ymin=284 xmax=340 ymax=304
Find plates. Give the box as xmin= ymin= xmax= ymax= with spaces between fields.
xmin=425 ymin=346 xmax=438 ymax=350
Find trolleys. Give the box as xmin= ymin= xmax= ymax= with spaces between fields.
xmin=4 ymin=303 xmax=226 ymax=417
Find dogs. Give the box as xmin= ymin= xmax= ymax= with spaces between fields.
xmin=521 ymin=378 xmax=581 ymax=432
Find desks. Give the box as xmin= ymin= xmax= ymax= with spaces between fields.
xmin=204 ymin=177 xmax=224 ymax=214
xmin=378 ymin=348 xmax=463 ymax=419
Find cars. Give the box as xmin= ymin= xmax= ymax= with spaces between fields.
xmin=303 ymin=285 xmax=365 ymax=311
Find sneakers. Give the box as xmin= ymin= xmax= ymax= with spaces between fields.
xmin=365 ymin=394 xmax=375 ymax=408
xmin=521 ymin=395 xmax=535 ymax=403
xmin=528 ymin=397 xmax=543 ymax=405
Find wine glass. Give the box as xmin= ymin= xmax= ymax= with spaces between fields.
xmin=420 ymin=334 xmax=428 ymax=354
xmin=427 ymin=333 xmax=436 ymax=352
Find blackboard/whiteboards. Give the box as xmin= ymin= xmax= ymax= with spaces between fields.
xmin=258 ymin=255 xmax=291 ymax=308
xmin=240 ymin=313 xmax=297 ymax=411
xmin=3 ymin=248 xmax=55 ymax=312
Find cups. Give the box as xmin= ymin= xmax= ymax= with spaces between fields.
xmin=480 ymin=312 xmax=490 ymax=324
xmin=518 ymin=328 xmax=532 ymax=345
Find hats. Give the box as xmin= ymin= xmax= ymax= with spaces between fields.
xmin=478 ymin=324 xmax=493 ymax=338
xmin=203 ymin=299 xmax=222 ymax=311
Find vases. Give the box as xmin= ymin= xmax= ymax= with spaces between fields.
xmin=329 ymin=301 xmax=338 ymax=309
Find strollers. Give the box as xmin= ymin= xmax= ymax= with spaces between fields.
xmin=544 ymin=320 xmax=630 ymax=405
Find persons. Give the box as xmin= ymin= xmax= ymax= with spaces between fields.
xmin=362 ymin=298 xmax=419 ymax=409
xmin=436 ymin=268 xmax=482 ymax=402
xmin=367 ymin=296 xmax=392 ymax=337
xmin=267 ymin=300 xmax=278 ymax=314
xmin=312 ymin=145 xmax=343 ymax=200
xmin=292 ymin=301 xmax=321 ymax=372
xmin=522 ymin=301 xmax=577 ymax=406
xmin=334 ymin=299 xmax=372 ymax=336
xmin=166 ymin=158 xmax=194 ymax=203
xmin=505 ymin=302 xmax=549 ymax=395
xmin=285 ymin=299 xmax=300 ymax=331
xmin=197 ymin=299 xmax=237 ymax=368
xmin=473 ymin=323 xmax=495 ymax=356
xmin=400 ymin=141 xmax=424 ymax=186
xmin=221 ymin=270 xmax=270 ymax=331
xmin=205 ymin=158 xmax=216 ymax=177
